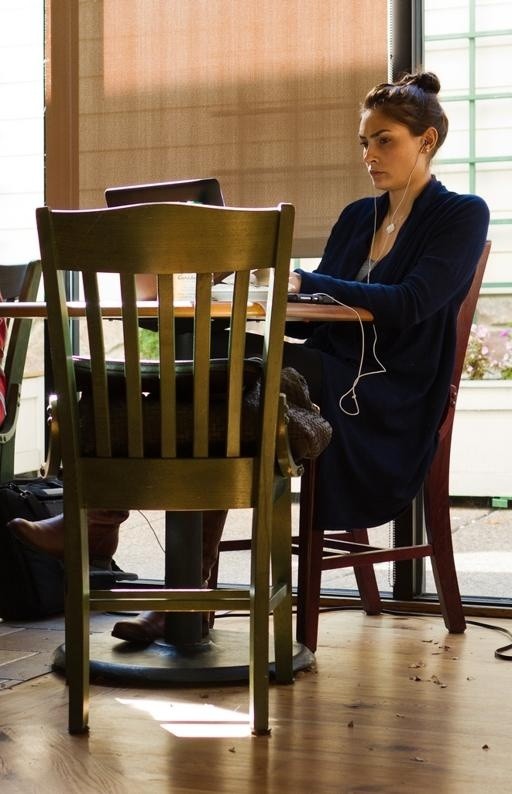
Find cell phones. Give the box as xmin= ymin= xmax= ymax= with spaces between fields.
xmin=288 ymin=292 xmax=335 ymax=303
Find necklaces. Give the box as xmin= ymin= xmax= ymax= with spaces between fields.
xmin=385 ymin=204 xmax=410 ymax=235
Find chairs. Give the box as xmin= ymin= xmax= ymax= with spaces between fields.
xmin=199 ymin=237 xmax=493 ymax=649
xmin=33 ymin=204 xmax=300 ymax=740
xmin=0 ymin=258 xmax=40 ymax=523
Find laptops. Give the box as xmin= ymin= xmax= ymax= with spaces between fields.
xmin=103 ymin=178 xmax=235 ymax=301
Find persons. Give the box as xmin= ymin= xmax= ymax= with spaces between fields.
xmin=8 ymin=71 xmax=490 ymax=650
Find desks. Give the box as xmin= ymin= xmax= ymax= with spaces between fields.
xmin=1 ymin=294 xmax=377 ymax=689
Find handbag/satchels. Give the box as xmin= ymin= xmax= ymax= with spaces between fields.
xmin=0 ymin=476 xmax=119 ymax=621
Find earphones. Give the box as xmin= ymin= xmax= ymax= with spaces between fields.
xmin=424 ymin=140 xmax=429 ymax=145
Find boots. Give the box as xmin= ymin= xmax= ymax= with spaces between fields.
xmin=4 ymin=507 xmax=128 ymax=569
xmin=112 ymin=512 xmax=227 ymax=644
